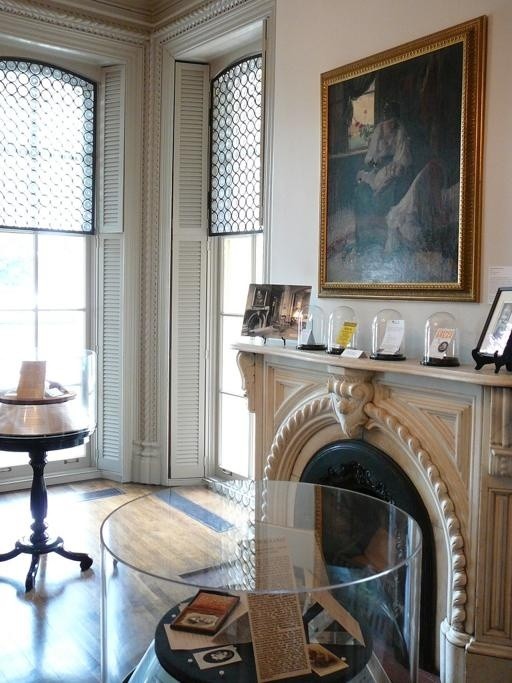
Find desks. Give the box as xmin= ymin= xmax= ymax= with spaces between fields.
xmin=153 ymin=587 xmax=377 ymax=681
xmin=1 ymin=427 xmax=96 ymax=592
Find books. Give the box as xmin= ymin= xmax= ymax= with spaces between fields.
xmin=170 ymin=588 xmax=240 ymax=635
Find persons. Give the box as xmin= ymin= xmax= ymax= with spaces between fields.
xmin=353 ymin=100 xmax=413 ymax=251
xmin=204 ymin=649 xmax=234 ymax=662
xmin=186 ymin=615 xmax=214 ymax=624
xmin=383 ymin=137 xmax=452 ymax=259
xmin=308 ymin=644 xmax=337 ymax=669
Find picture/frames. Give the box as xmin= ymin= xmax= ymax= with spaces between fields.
xmin=473 ymin=287 xmax=510 ymax=360
xmin=315 ymin=14 xmax=488 ymax=303
